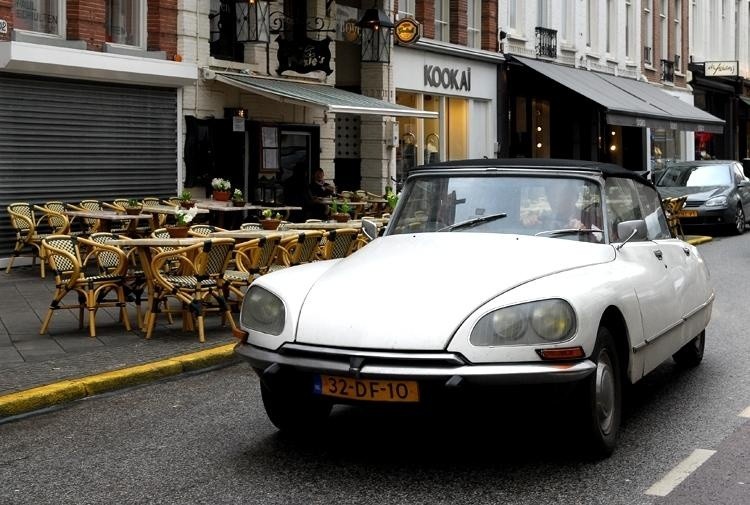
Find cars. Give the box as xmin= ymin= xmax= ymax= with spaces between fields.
xmin=233 ymin=159 xmax=716 ymax=458
xmin=654 ymin=158 xmax=749 ymax=234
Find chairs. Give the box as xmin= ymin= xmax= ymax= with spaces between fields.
xmin=89 ymin=232 xmax=174 ymax=328
xmin=189 ymin=191 xmax=391 ymax=280
xmin=114 ymin=198 xmax=144 ymax=223
xmin=220 ymin=234 xmax=282 ymax=327
xmin=662 ymin=194 xmax=687 ymax=237
xmin=142 ymin=198 xmax=175 ymax=224
xmin=6 ymin=202 xmax=68 ymax=278
xmin=168 ymin=197 xmax=188 ymax=220
xmin=151 ymin=229 xmax=186 ymax=269
xmin=44 ymin=201 xmax=88 ymax=234
xmin=144 ymin=238 xmax=239 ymax=344
xmin=39 ymin=235 xmax=131 ymax=338
xmin=80 ymin=200 xmax=124 ymax=232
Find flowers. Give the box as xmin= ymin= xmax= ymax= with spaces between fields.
xmin=211 ymin=178 xmax=231 ymax=191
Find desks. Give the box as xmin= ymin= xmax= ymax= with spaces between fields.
xmin=64 ymin=210 xmax=152 ymax=233
xmin=106 ymin=237 xmax=236 ymax=332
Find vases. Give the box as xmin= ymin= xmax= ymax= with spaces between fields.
xmin=213 ymin=191 xmax=229 ymax=202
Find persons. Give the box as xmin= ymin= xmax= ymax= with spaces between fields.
xmin=303 ymin=167 xmax=335 ymax=221
xmin=525 ymin=176 xmax=593 ymax=243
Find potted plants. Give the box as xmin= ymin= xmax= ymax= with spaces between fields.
xmin=126 ymin=197 xmax=142 ymax=215
xmin=181 ymin=192 xmax=191 ymax=208
xmin=167 ymin=213 xmax=188 ymax=238
